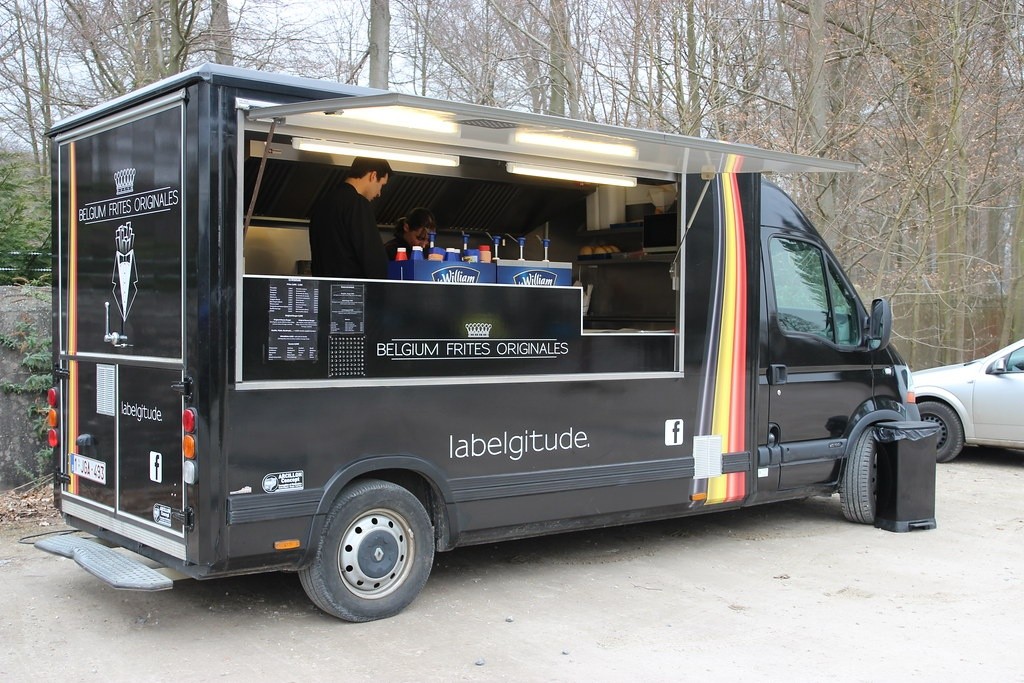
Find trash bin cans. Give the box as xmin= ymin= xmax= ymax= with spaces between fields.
xmin=871 ymin=420 xmax=939 ymax=534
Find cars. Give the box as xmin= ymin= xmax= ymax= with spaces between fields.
xmin=910 ymin=338 xmax=1024 ymax=464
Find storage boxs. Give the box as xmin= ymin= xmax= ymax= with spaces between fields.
xmin=586 ymin=187 xmax=600 ymax=231
xmin=598 ymin=185 xmax=626 ymax=231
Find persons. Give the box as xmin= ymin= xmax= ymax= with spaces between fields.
xmin=386 ymin=207 xmax=439 ymax=259
xmin=309 ymin=156 xmax=392 ymax=279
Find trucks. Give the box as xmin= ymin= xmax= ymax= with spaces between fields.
xmin=34 ymin=62 xmax=924 ymax=624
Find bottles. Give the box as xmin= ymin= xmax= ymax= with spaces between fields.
xmin=396 ymin=248 xmax=406 ymax=260
xmin=479 ymin=245 xmax=491 ymax=263
xmin=446 ymin=248 xmax=456 ymax=261
xmin=410 ymin=246 xmax=425 ymax=260
xmin=455 ymin=249 xmax=461 ymax=261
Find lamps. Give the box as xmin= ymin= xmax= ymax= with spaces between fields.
xmin=291 ymin=136 xmax=460 ymax=167
xmin=506 ymin=162 xmax=637 ymax=187
